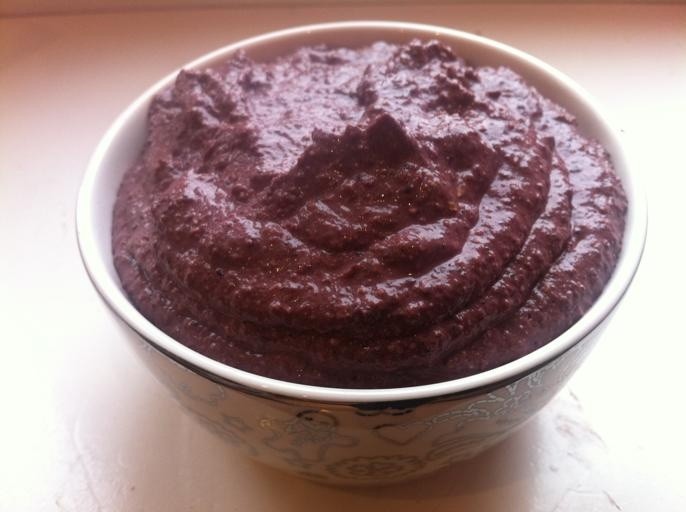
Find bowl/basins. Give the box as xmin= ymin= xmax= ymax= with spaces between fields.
xmin=73 ymin=16 xmax=651 ymax=487
